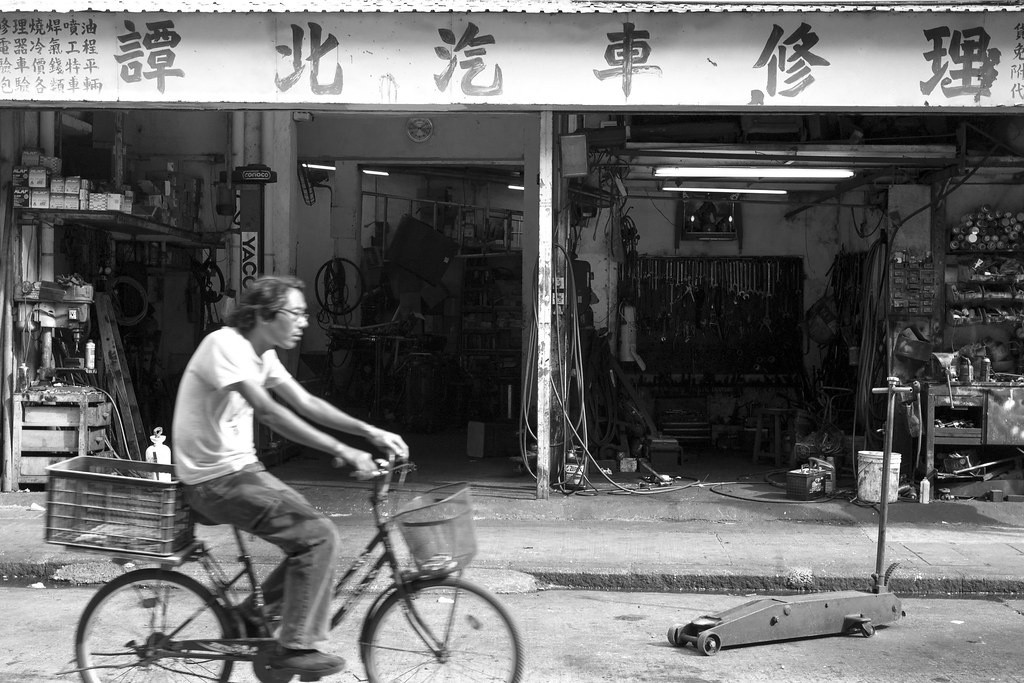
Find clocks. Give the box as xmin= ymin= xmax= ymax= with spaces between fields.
xmin=404 ymin=117 xmax=434 ymax=143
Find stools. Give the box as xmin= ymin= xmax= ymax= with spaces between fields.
xmin=753 ymin=408 xmax=797 ymax=469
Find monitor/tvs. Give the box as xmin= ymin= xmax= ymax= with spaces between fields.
xmin=681 ymin=198 xmax=737 ymax=241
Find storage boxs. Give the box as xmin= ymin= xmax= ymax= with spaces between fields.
xmin=65 ymin=195 xmax=80 ymax=211
xmin=50 ymin=194 xmax=65 ymax=210
xmin=90 ymin=193 xmax=107 ymax=211
xmin=50 ymin=177 xmax=65 ymax=194
xmin=124 ymin=157 xmax=201 ymax=232
xmin=65 ymin=176 xmax=81 ymax=195
xmin=79 ymin=189 xmax=90 ymax=200
xmin=11 ymin=165 xmax=31 ymax=187
xmin=44 ymin=455 xmax=196 ymax=558
xmin=29 ymin=166 xmax=52 ymax=189
xmin=390 ymin=215 xmax=461 ymax=288
xmin=13 ymin=187 xmax=32 ymax=209
xmin=82 ymin=179 xmax=96 ymax=191
xmin=945 ymin=262 xmax=1024 ymax=328
xmin=40 ymin=155 xmax=62 ymax=174
xmin=106 ymin=193 xmax=125 ymax=211
xmin=22 ymin=151 xmax=44 ymax=166
xmin=80 ymin=200 xmax=89 ymax=210
xmin=31 ymin=189 xmax=50 ymax=209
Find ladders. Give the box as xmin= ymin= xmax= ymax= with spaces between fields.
xmin=93 ymin=290 xmax=147 ymax=462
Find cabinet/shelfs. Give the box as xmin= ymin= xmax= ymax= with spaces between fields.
xmin=929 ymin=162 xmax=1024 ymax=328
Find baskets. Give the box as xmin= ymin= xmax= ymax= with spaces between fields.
xmin=392 ymin=480 xmax=478 ymax=576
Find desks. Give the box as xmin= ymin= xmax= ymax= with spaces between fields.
xmin=908 ymin=377 xmax=1024 ymax=500
xmin=12 ymin=390 xmax=114 ymax=498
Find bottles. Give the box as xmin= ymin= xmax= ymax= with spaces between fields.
xmin=950 ymin=205 xmax=1024 ymax=251
xmin=980 ymin=356 xmax=991 ymax=382
xmin=919 ymin=477 xmax=930 ymax=504
xmin=86 ymin=340 xmax=95 ymax=369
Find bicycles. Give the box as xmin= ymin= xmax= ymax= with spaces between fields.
xmin=55 ymin=448 xmax=523 ymax=683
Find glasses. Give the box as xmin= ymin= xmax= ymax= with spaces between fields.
xmin=279 ymin=308 xmax=310 ymax=321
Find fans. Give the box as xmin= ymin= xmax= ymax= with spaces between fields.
xmin=297 ymin=159 xmax=332 ymax=206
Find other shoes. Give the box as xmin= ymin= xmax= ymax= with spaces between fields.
xmin=265 ymin=650 xmax=346 ymax=677
xmin=237 ymin=603 xmax=272 ymax=638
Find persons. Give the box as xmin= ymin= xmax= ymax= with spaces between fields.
xmin=172 ymin=275 xmax=410 ymax=677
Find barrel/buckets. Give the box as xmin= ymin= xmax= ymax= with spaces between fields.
xmin=857 ymin=450 xmax=901 ymax=503
